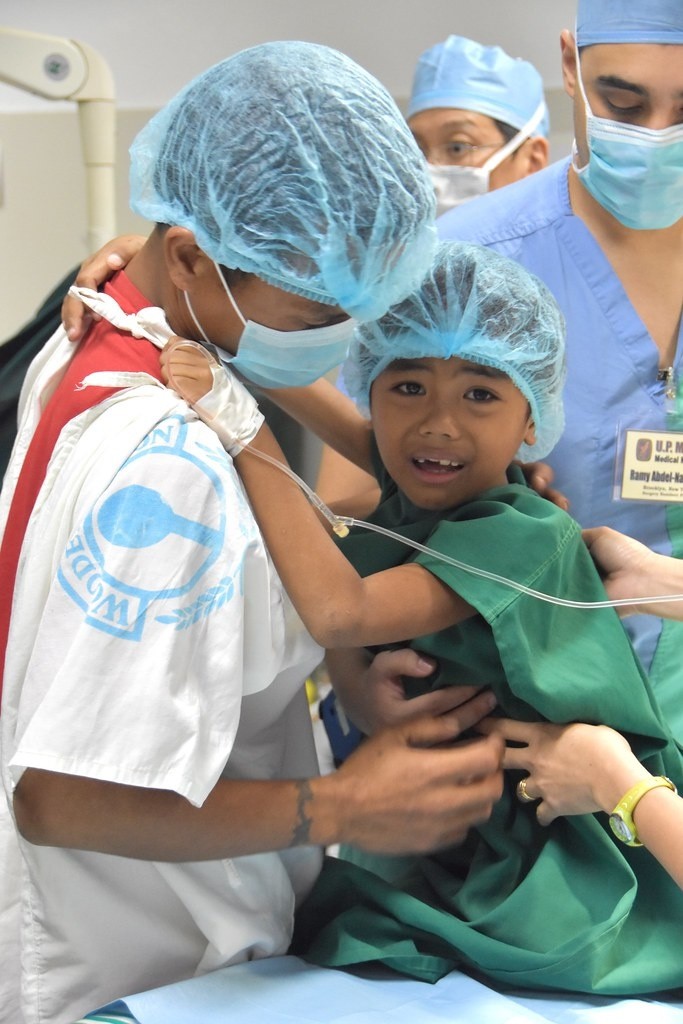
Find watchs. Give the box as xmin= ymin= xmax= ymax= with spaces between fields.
xmin=607 ymin=775 xmax=676 ymax=846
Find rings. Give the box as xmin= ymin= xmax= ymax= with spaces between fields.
xmin=518 ymin=779 xmax=536 ymax=801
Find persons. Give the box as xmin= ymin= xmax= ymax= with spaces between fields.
xmin=309 ymin=0 xmax=683 ymax=679
xmin=476 ymin=527 xmax=683 ymax=892
xmin=60 ymin=231 xmax=683 ymax=999
xmin=1 ymin=40 xmax=504 ymax=1024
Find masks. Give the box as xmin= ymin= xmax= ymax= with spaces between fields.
xmin=184 ymin=262 xmax=355 ymax=391
xmin=426 ymin=98 xmax=548 ymax=221
xmin=567 ymin=53 xmax=681 ymax=231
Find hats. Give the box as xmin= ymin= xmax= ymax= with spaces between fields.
xmin=404 ymin=34 xmax=551 ymax=142
xmin=574 ymin=1 xmax=682 ymax=49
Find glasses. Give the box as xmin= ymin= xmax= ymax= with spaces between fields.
xmin=411 ymin=140 xmax=499 ymax=167
